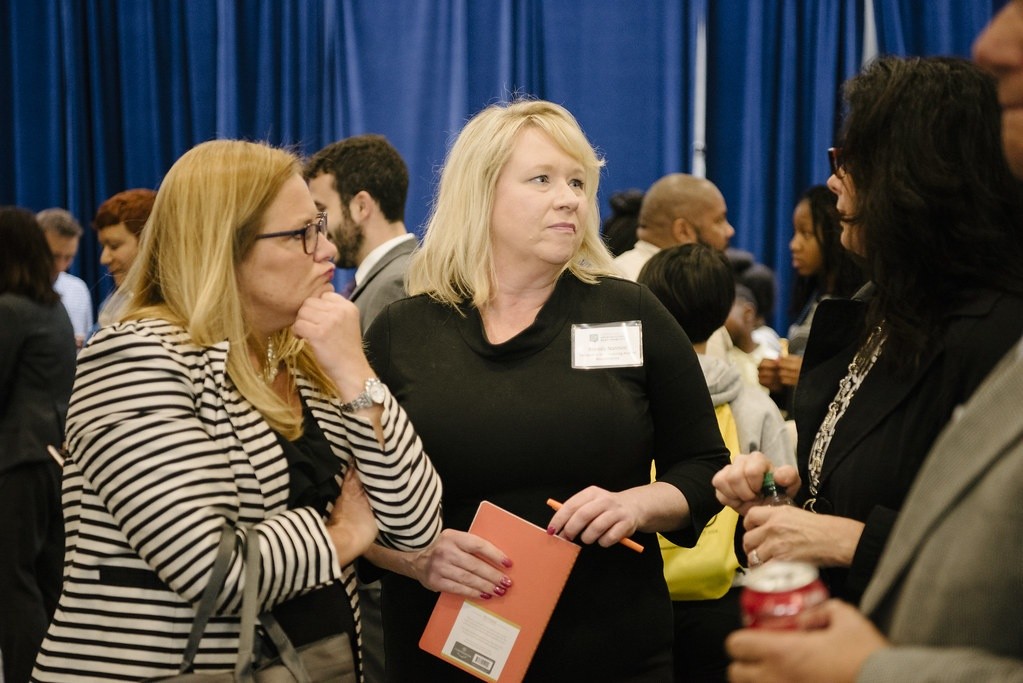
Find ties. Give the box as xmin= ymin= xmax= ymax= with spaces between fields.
xmin=341 ymin=277 xmax=359 ymax=300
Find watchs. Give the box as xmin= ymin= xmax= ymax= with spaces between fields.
xmin=340 ymin=378 xmax=385 ymax=413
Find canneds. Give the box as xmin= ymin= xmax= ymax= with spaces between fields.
xmin=739 ymin=560 xmax=829 ymax=631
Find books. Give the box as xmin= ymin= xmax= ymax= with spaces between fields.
xmin=419 ymin=501 xmax=581 ymax=683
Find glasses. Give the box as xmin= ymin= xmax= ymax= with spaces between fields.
xmin=252 ymin=211 xmax=330 ymax=253
xmin=828 ymin=148 xmax=848 ymax=179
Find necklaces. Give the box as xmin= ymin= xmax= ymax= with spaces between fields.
xmin=257 ymin=337 xmax=280 ymax=383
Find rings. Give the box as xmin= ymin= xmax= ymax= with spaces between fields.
xmin=752 ymin=550 xmax=764 ymax=565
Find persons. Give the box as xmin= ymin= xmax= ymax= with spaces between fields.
xmin=602 ymin=173 xmax=783 ymax=365
xmin=636 ymin=241 xmax=798 ymax=683
xmin=29 ymin=139 xmax=442 ymax=683
xmin=726 ymin=0 xmax=1023 ymax=683
xmin=362 ymin=102 xmax=731 ymax=683
xmin=303 ymin=133 xmax=421 ymax=334
xmin=709 ymin=52 xmax=1023 ymax=607
xmin=0 ymin=205 xmax=78 ymax=683
xmin=757 ymin=183 xmax=864 ymax=400
xmin=35 ymin=207 xmax=93 ymax=348
xmin=92 ymin=188 xmax=157 ymax=286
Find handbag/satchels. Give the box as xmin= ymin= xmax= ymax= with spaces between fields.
xmin=161 ymin=524 xmax=356 ymax=683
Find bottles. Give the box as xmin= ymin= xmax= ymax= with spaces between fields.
xmin=761 ymin=469 xmax=796 ymax=509
